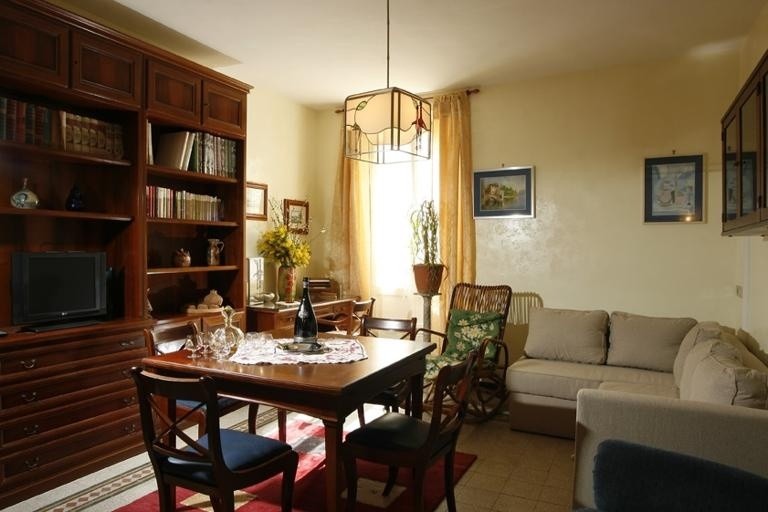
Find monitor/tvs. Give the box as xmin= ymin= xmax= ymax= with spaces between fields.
xmin=12 ymin=251 xmax=107 ymax=333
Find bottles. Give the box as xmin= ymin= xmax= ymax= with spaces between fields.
xmin=66 ymin=185 xmax=86 ymax=211
xmin=294 ymin=277 xmax=319 ymax=343
xmin=216 ymin=307 xmax=245 ymax=352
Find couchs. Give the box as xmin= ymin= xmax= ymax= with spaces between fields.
xmin=571 ymin=351 xmax=768 ymax=512
xmin=505 ymin=329 xmax=749 ymax=442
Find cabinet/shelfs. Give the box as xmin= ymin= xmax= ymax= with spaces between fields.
xmin=0 ymin=0 xmax=256 ymax=511
xmin=720 ymin=48 xmax=768 ymax=236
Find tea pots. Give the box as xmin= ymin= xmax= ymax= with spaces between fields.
xmin=207 ymin=239 xmax=224 ymax=265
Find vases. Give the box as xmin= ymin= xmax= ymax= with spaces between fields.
xmin=277 ymin=266 xmax=298 ymax=303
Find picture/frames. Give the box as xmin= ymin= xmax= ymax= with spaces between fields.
xmin=245 ymin=181 xmax=268 ymax=222
xmin=283 ymin=198 xmax=310 ymax=235
xmin=472 ymin=166 xmax=536 ymax=219
xmin=643 ymin=152 xmax=706 ymax=225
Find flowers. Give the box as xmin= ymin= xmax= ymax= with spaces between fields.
xmin=255 ymin=194 xmax=331 ymax=270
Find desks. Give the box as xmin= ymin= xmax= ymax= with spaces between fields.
xmin=250 ymin=297 xmax=354 ymax=335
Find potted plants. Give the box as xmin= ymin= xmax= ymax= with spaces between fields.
xmin=409 ymin=198 xmax=450 ymax=296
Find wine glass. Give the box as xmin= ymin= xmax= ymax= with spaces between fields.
xmin=186 ymin=331 xmax=234 ymax=364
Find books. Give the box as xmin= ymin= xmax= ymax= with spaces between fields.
xmin=146 ymin=118 xmax=238 ymax=179
xmin=0 ymin=96 xmax=129 ymax=160
xmin=146 ymin=185 xmax=226 ymax=223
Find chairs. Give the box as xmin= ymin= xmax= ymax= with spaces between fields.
xmin=143 ymin=320 xmax=258 ymax=449
xmin=130 ymin=366 xmax=298 ymax=512
xmin=345 ymin=351 xmax=477 ymax=512
xmin=400 ymin=283 xmax=512 ymax=425
xmin=359 ymin=314 xmax=417 ymax=412
xmin=317 ymin=298 xmax=375 ymax=334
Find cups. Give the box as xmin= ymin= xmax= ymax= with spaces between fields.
xmin=246 ymin=331 xmax=273 ymax=357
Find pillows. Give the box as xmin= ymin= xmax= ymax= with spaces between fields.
xmin=524 ymin=306 xmax=768 ymax=410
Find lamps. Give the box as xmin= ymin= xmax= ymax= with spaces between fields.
xmin=344 ymin=0 xmax=431 ymax=166
